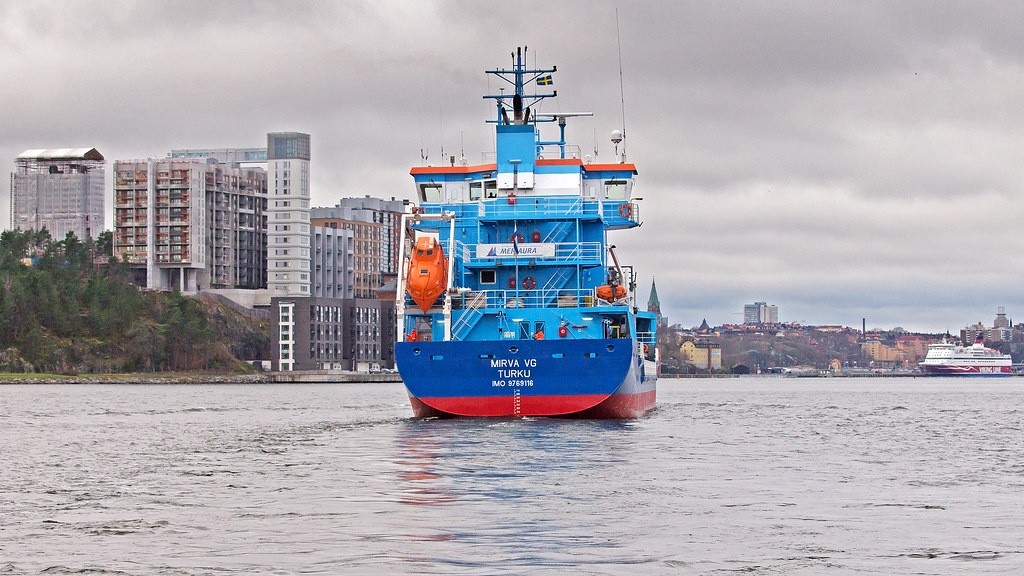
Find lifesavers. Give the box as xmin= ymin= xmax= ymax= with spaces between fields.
xmin=523 ymin=276 xmax=536 ymax=290
xmin=619 ymin=204 xmax=629 ymax=218
xmin=413 ymin=208 xmax=424 ymax=214
xmin=511 ymin=233 xmax=524 ymax=243
xmin=643 ymin=344 xmax=649 ymax=353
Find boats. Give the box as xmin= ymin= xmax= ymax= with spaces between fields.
xmin=388 ymin=45 xmax=663 ymax=423
xmin=918 ymin=335 xmax=1013 ymax=375
